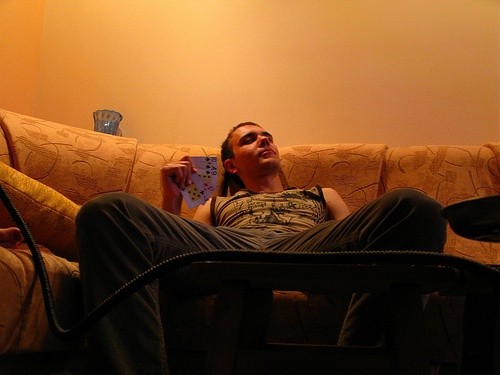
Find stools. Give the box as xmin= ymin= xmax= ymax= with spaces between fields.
xmin=192 ymin=259 xmax=461 ymax=375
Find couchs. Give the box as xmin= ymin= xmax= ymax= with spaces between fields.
xmin=0 ymin=109 xmax=500 ymax=375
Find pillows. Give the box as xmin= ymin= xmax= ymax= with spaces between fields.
xmin=0 ymin=161 xmax=80 ymax=258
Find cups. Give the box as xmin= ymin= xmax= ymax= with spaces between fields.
xmin=92 ymin=109 xmax=123 ymax=135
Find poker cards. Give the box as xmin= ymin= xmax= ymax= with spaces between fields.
xmin=178 ymin=157 xmax=218 ymax=209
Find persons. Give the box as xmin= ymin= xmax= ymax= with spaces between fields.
xmin=77 ymin=122 xmax=447 ymax=375
xmin=0 ymin=227 xmax=24 ymax=249
